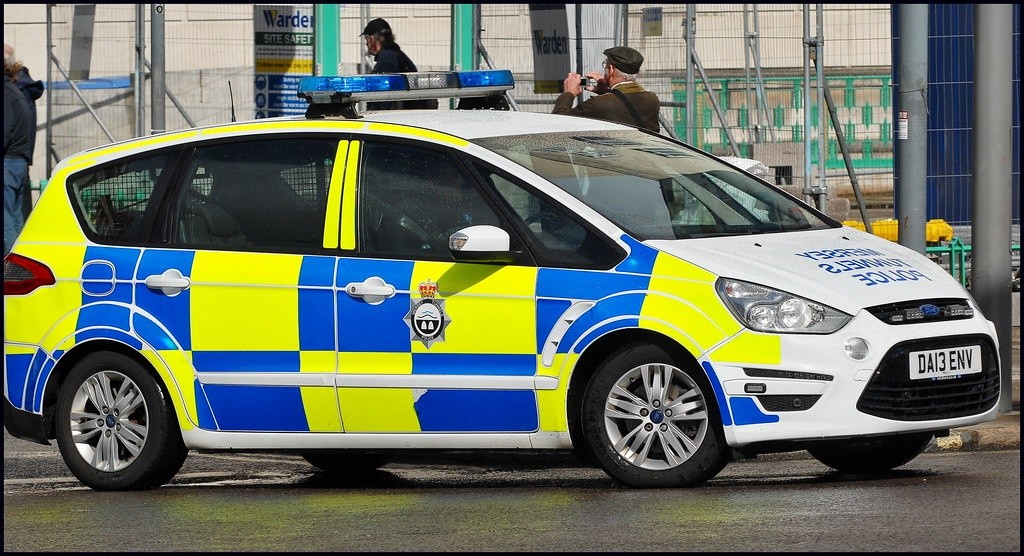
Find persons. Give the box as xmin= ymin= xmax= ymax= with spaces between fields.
xmin=552 ymin=46 xmax=662 ymax=228
xmin=359 ymin=17 xmax=428 ymax=111
xmin=4 ymin=44 xmax=45 ymax=257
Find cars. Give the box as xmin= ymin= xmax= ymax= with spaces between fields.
xmin=2 ymin=66 xmax=1005 ymax=491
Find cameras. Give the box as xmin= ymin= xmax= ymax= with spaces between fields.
xmin=579 ymin=77 xmax=589 ymax=87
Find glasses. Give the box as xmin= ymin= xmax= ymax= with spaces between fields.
xmin=602 ymin=61 xmax=612 ymax=69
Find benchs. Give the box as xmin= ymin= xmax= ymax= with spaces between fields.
xmin=181 ymin=172 xmax=321 ymax=251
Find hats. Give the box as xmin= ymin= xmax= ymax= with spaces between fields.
xmin=603 ymin=47 xmax=644 ymax=74
xmin=359 ymin=18 xmax=391 ymax=36
